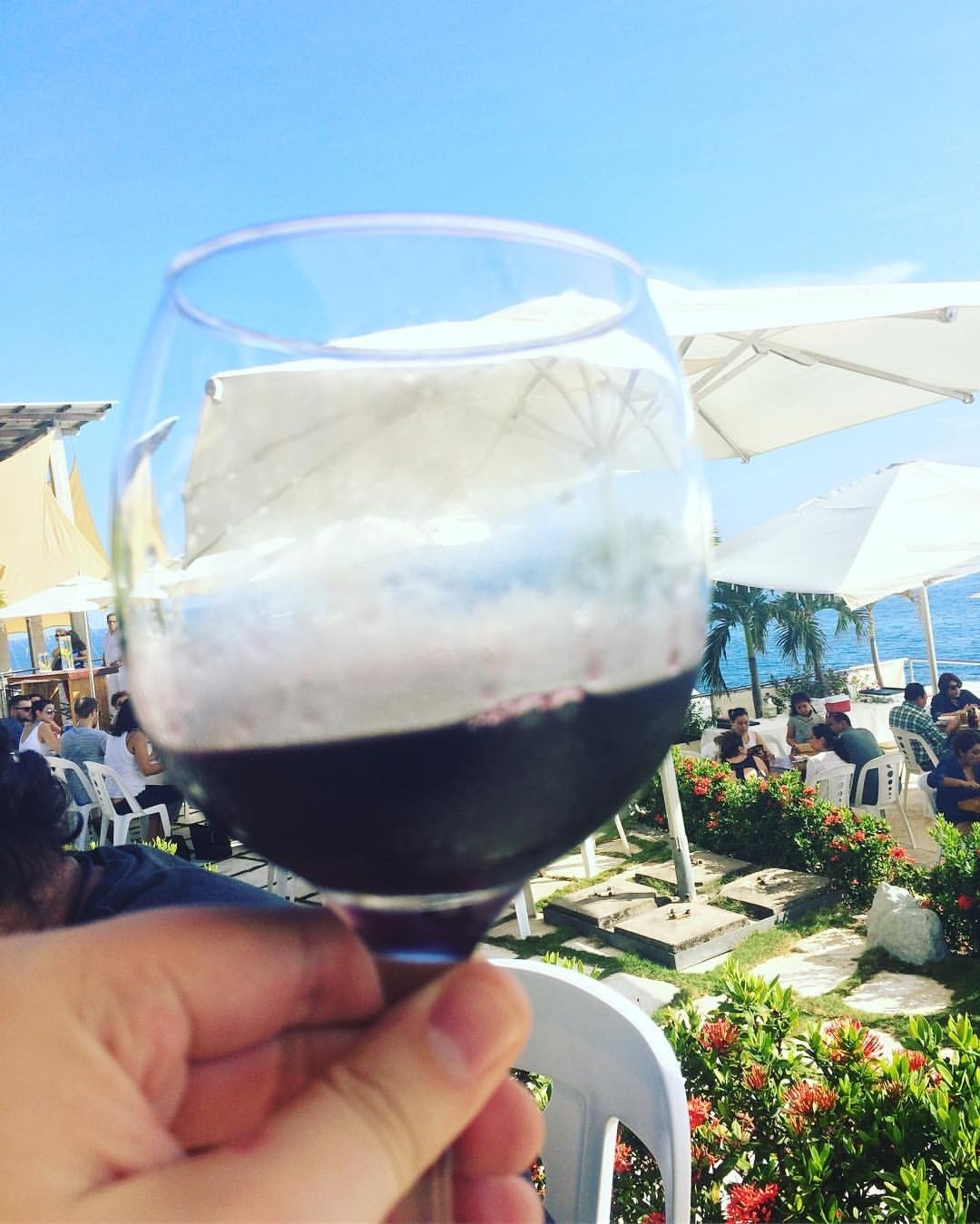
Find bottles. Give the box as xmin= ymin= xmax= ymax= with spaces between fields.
xmin=65 ymin=718 xmax=72 ymax=732
xmin=147 ymin=741 xmax=153 ymax=756
xmin=968 ymin=708 xmax=975 ymax=728
xmin=763 ymin=751 xmax=770 ymax=771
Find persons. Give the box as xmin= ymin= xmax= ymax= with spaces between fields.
xmin=805 ymin=723 xmax=849 ymax=787
xmin=8 ymin=691 xmax=184 ymax=844
xmin=51 ymin=628 xmax=88 ymax=701
xmin=786 ymin=692 xmax=825 ymax=755
xmin=102 ymin=613 xmax=125 ymax=712
xmin=930 ymin=673 xmax=980 ymax=722
xmin=890 ymin=683 xmax=959 ymax=772
xmin=0 ymin=907 xmax=544 ymax=1224
xmin=928 ymin=731 xmax=980 ymax=828
xmin=0 ymin=751 xmax=296 ymax=935
xmin=827 ymin=711 xmax=902 ymax=806
xmin=715 ymin=707 xmax=768 ymax=782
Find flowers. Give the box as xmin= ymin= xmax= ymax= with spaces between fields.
xmin=776 ymin=665 xmax=864 ymax=697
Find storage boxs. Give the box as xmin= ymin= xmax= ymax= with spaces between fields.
xmin=825 ymin=695 xmax=851 ymax=714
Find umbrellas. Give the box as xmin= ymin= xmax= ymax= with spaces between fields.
xmin=189 ymin=276 xmax=980 ymax=909
xmin=713 ymin=459 xmax=980 ymax=696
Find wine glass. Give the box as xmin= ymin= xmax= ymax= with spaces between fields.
xmin=109 ymin=213 xmax=711 ymax=1223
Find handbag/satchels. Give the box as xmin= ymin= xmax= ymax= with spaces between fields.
xmin=188 ymin=822 xmax=232 ymax=860
xmin=164 ymin=834 xmax=191 ymax=862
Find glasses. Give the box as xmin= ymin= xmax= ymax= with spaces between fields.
xmin=949 ymin=682 xmax=962 ymax=690
xmin=55 ymin=633 xmax=65 ymax=637
xmin=40 ymin=708 xmax=57 ymax=715
xmin=14 ymin=706 xmax=32 ymax=711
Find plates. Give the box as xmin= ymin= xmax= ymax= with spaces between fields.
xmin=937 ymin=716 xmax=957 ymax=722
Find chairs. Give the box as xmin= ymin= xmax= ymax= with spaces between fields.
xmin=41 ymin=756 xmax=109 ymax=850
xmin=83 ymin=760 xmax=170 ymax=849
xmin=807 ymin=724 xmax=943 ymax=850
xmin=479 ymin=954 xmax=692 ymax=1223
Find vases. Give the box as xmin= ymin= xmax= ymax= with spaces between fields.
xmin=807 ymin=697 xmax=828 ymax=717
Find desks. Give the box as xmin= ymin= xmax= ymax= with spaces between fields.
xmin=699 ymin=678 xmax=975 ymax=760
xmin=935 ymin=706 xmax=980 ymax=731
xmin=763 ymin=750 xmax=817 ymax=772
